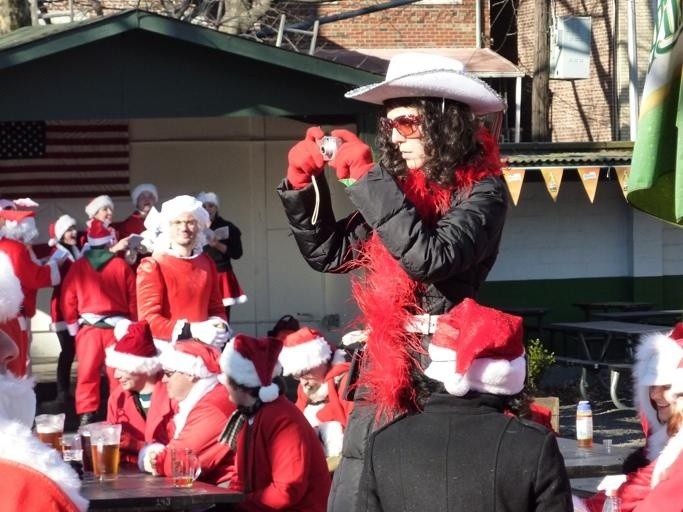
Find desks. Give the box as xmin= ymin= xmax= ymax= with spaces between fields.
xmin=489 ymin=301 xmax=683 ymax=361
xmin=56 ymin=425 xmax=243 ymax=512
xmin=548 ymin=428 xmax=640 ymax=477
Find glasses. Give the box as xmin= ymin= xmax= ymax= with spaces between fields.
xmin=379 ymin=115 xmax=426 ymax=137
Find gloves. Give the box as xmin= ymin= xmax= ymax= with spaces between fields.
xmin=287 ymin=126 xmax=326 ymax=189
xmin=326 ymin=129 xmax=378 ymax=187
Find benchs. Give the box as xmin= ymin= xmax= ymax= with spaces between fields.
xmin=569 ymin=473 xmax=631 ymax=499
xmin=548 ymin=354 xmax=635 ymax=410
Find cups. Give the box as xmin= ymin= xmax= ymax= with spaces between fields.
xmin=59 ymin=435 xmax=85 ymax=481
xmin=33 ymin=413 xmax=67 ymax=457
xmin=169 ymin=448 xmax=200 ymax=491
xmin=88 ymin=424 xmax=121 ymax=484
xmin=599 ymin=495 xmax=620 ymax=512
xmin=601 ymin=439 xmax=612 ymax=456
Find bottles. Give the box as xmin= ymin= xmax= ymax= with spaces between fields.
xmin=574 ymin=399 xmax=595 ymax=449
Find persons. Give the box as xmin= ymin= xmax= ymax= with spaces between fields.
xmin=1 ymin=184 xmax=366 ymax=512
xmin=277 ymin=50 xmax=509 ymax=511
xmin=357 ymin=302 xmax=575 ymax=512
xmin=565 ymin=320 xmax=683 ymax=512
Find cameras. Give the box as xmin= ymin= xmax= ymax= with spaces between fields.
xmin=315 ymin=136 xmax=342 ymax=161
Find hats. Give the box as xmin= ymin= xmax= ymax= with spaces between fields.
xmin=105 ymin=319 xmax=348 ymax=403
xmin=644 ymin=354 xmax=672 ymax=385
xmin=344 ymin=52 xmax=509 ymax=116
xmin=424 ymin=297 xmax=526 ymax=397
xmin=0 ymin=183 xmax=218 ymax=254
